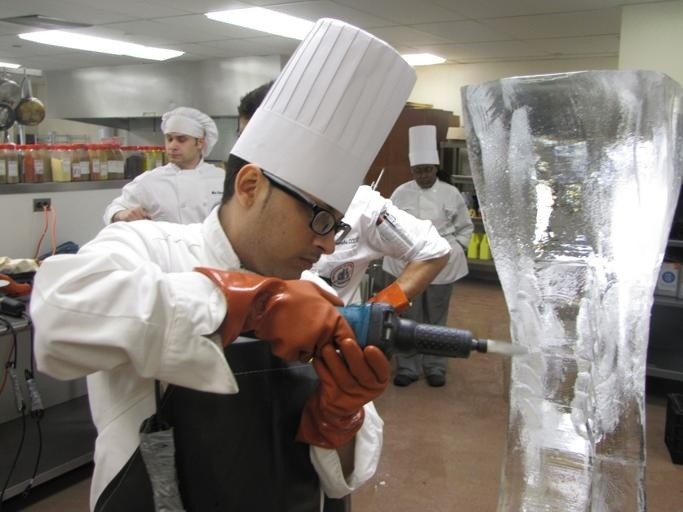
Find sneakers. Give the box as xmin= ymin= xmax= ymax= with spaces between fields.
xmin=426 ymin=375 xmax=446 ymax=386
xmin=394 ymin=374 xmax=418 ymax=387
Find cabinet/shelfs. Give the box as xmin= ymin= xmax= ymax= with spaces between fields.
xmin=437 ymin=139 xmax=496 ymax=266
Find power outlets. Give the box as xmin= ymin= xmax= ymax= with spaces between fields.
xmin=32 ymin=198 xmax=50 ymax=213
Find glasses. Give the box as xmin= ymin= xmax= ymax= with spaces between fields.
xmin=262 ymin=171 xmax=352 ymax=245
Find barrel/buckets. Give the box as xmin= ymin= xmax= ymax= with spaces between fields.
xmin=479 ymin=234 xmax=493 ymax=261
xmin=467 ymin=232 xmax=482 ymax=260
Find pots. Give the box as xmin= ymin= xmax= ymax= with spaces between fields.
xmin=0 ymin=73 xmax=45 ymax=132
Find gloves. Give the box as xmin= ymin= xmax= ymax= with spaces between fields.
xmin=294 ymin=337 xmax=394 ymax=450
xmin=193 ymin=267 xmax=356 ymax=364
xmin=367 ymin=282 xmax=413 ymax=316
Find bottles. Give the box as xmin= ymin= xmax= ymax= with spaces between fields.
xmin=0 ymin=143 xmax=166 ymax=185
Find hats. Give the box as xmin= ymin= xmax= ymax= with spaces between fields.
xmin=160 ymin=106 xmax=218 ymax=158
xmin=408 ymin=124 xmax=439 ymax=167
xmin=229 ymin=17 xmax=417 ymax=216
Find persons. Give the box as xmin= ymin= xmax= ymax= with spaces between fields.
xmin=27 ymin=16 xmax=417 ymax=511
xmin=382 ymin=125 xmax=475 ymax=387
xmin=235 ymin=79 xmax=453 ymax=511
xmin=102 ymin=106 xmax=227 ymax=226
